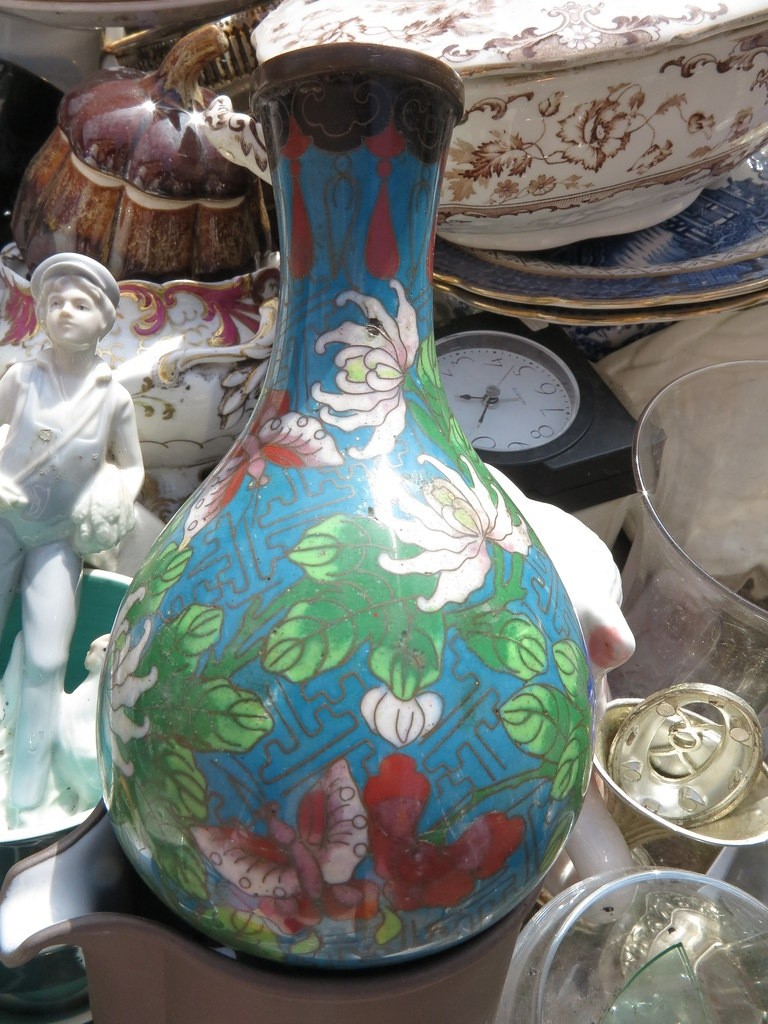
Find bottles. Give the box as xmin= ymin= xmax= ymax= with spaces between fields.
xmin=495 ymin=869 xmax=767 ymax=1023
xmin=95 ymin=41 xmax=593 ymax=975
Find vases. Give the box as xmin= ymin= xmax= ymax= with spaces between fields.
xmin=96 ymin=43 xmax=591 ymax=972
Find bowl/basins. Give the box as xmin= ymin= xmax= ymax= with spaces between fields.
xmin=251 ymin=0 xmax=768 ymax=368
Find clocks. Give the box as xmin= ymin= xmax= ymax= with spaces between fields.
xmin=433 ymin=331 xmax=596 ymax=468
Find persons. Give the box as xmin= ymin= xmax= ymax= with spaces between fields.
xmin=0 ymin=253 xmax=144 ymax=813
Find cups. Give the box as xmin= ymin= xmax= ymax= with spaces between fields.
xmin=607 ymin=358 xmax=768 ymax=739
